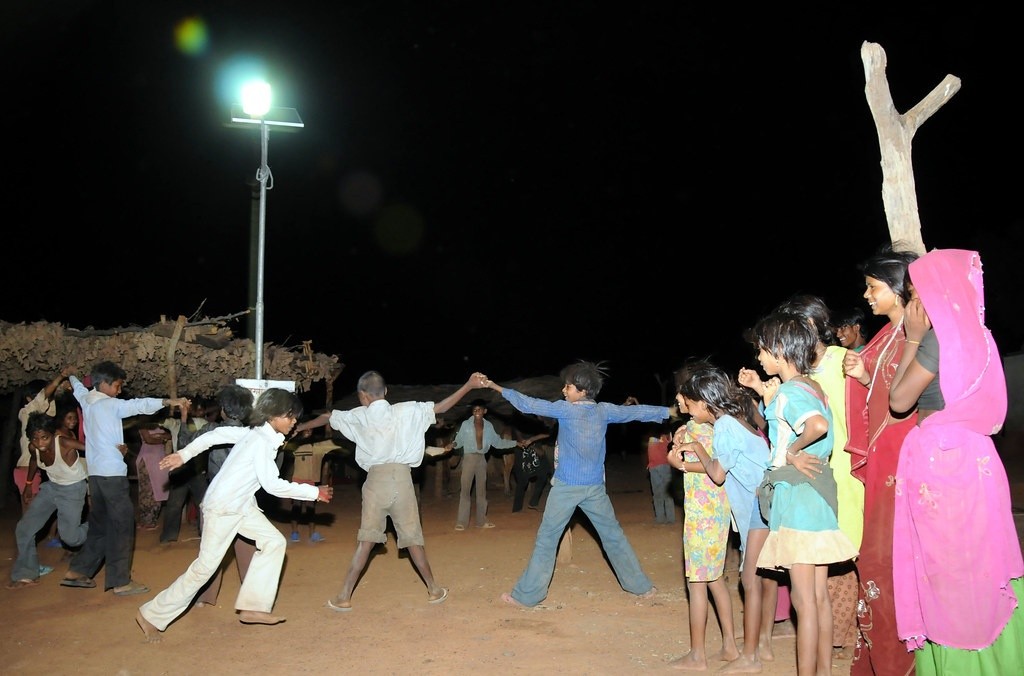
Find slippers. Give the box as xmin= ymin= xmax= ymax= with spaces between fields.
xmin=6 ymin=578 xmax=40 ymax=589
xmin=60 ymin=575 xmax=97 ymax=588
xmin=321 ymin=600 xmax=352 ymax=611
xmin=429 ymin=588 xmax=449 ymax=604
xmin=118 ymin=582 xmax=150 ymax=595
xmin=455 ymin=525 xmax=465 ymax=530
xmin=473 ymin=522 xmax=496 ymax=529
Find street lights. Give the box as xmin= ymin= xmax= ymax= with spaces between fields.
xmin=222 ymin=80 xmax=305 ymax=407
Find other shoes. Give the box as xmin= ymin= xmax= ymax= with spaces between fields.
xmin=290 ymin=532 xmax=299 ymax=541
xmin=512 ymin=510 xmax=526 ymax=514
xmin=38 ymin=565 xmax=54 ymax=576
xmin=44 ymin=538 xmax=62 ymax=548
xmin=309 ymin=532 xmax=325 ymax=542
xmin=640 ymin=587 xmax=657 ymax=599
xmin=528 ymin=505 xmax=544 ymax=512
xmin=501 ymin=593 xmax=538 ymax=612
xmin=159 ymin=538 xmax=178 ymax=543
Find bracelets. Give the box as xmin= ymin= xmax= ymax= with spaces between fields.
xmin=785 ymin=449 xmax=802 ymax=457
xmin=678 ymin=462 xmax=687 ymax=473
xmin=904 ymin=340 xmax=921 ymax=344
xmin=25 ymin=481 xmax=32 ymax=485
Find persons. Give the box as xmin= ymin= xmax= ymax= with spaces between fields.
xmin=285 ymin=371 xmax=487 ymax=613
xmin=281 ymin=421 xmax=356 ymax=542
xmin=471 ymin=360 xmax=679 ymax=608
xmin=6 ymin=360 xmax=258 ymax=608
xmin=632 ymin=246 xmax=1024 ymax=676
xmin=136 ymin=388 xmax=333 ymax=646
xmin=422 ymin=396 xmax=632 ymax=530
